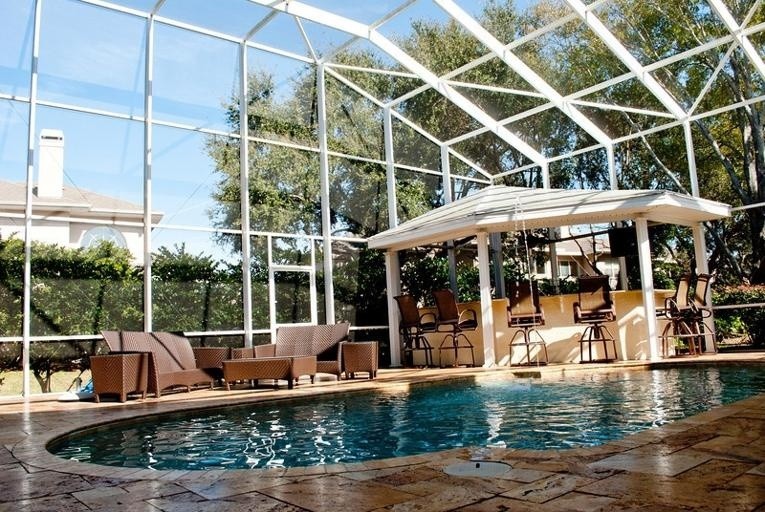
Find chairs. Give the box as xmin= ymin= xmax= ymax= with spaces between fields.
xmin=573 ymin=274 xmax=618 ymax=363
xmin=395 ymin=295 xmax=439 ymax=368
xmin=655 ymin=274 xmax=717 ymax=358
xmin=507 ymin=278 xmax=549 ymax=366
xmin=433 ymin=289 xmax=478 ymax=366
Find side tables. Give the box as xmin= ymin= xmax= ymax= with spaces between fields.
xmin=343 ymin=341 xmax=378 ymax=380
xmin=91 ymin=352 xmax=150 ymax=401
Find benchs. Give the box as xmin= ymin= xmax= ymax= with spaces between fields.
xmin=100 ymin=329 xmax=231 ymax=401
xmin=231 ymin=323 xmax=351 ymax=384
